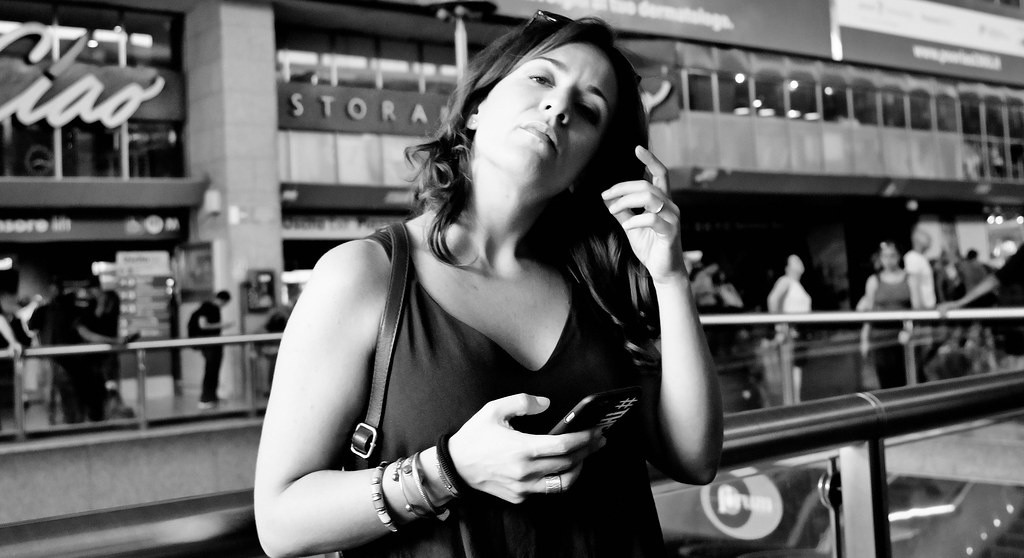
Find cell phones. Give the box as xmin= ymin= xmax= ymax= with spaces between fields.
xmin=546 ymin=384 xmax=647 ymax=439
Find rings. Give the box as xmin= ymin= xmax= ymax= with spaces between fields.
xmin=544 ymin=476 xmax=562 ymax=494
xmin=654 ymin=201 xmax=666 ymax=216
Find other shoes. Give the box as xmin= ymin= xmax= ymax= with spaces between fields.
xmin=198 ymin=401 xmax=215 ymax=410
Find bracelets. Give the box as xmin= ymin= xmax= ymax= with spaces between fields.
xmin=372 ymin=433 xmax=475 ymax=531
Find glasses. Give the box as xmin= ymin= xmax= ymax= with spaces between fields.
xmin=519 ymin=8 xmax=642 ymax=89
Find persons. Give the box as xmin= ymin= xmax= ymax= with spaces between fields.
xmin=252 ymin=11 xmax=722 ymax=558
xmin=0 ymin=239 xmax=1024 ymax=439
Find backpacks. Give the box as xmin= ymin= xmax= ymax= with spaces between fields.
xmin=187 ymin=308 xmax=203 ymax=349
